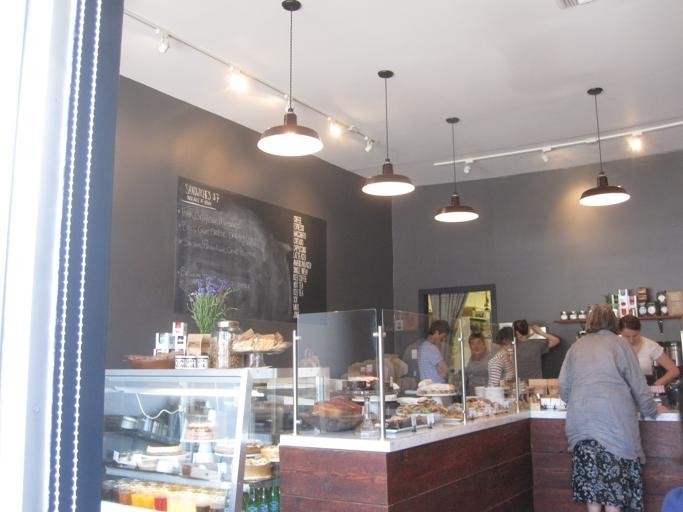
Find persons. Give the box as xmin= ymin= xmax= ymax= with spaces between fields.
xmin=488 ymin=327 xmax=514 ymax=388
xmin=513 ymin=319 xmax=560 ymax=380
xmin=466 ymin=332 xmax=487 ymax=388
xmin=416 ymin=320 xmax=450 ymax=386
xmin=557 ymin=305 xmax=668 ymax=512
xmin=617 ymin=313 xmax=680 ymax=389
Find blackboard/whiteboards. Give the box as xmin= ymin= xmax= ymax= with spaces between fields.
xmin=171 ymin=175 xmax=327 ymax=326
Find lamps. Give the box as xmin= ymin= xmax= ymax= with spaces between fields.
xmin=434 ymin=118 xmax=480 ymax=222
xmin=124 ymin=10 xmax=374 ymax=154
xmin=580 ymin=88 xmax=631 ymax=206
xmin=257 ymin=0 xmax=324 ymax=157
xmin=433 ymin=121 xmax=683 ymax=174
xmin=361 ymin=70 xmax=415 ymax=196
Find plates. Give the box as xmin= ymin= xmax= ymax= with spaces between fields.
xmin=438 ymin=418 xmax=463 ymax=425
xmin=385 ymin=424 xmax=425 ymax=433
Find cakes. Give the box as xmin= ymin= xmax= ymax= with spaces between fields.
xmin=258 ymin=445 xmax=279 ymax=460
xmin=416 ymin=381 xmax=456 ymax=394
xmin=244 ymin=459 xmax=271 ymax=478
xmin=242 ymin=440 xmax=262 ymax=452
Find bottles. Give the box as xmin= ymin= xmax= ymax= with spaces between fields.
xmin=243 ymin=486 xmax=280 ymax=512
xmin=212 ymin=320 xmax=247 ymax=368
xmin=578 ymin=311 xmax=588 ymax=319
xmin=570 ymin=310 xmax=578 ymax=321
xmin=559 ymin=310 xmax=569 ymax=320
xmin=224 ymin=490 xmax=230 ymax=512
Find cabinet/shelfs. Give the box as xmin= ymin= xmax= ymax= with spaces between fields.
xmin=100 ymin=365 xmax=330 ymax=512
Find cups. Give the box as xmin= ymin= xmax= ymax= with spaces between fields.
xmin=101 ymin=478 xmax=226 ymax=512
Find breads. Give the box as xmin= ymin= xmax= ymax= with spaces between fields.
xmin=314 ymin=396 xmax=362 ymax=419
xmin=232 ymin=329 xmax=289 ymax=352
